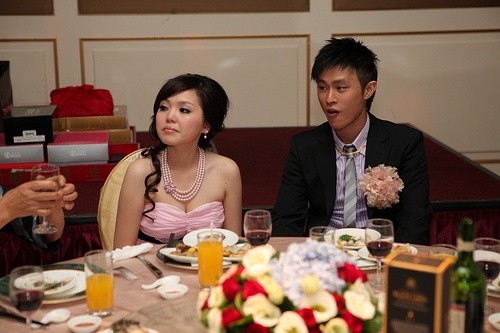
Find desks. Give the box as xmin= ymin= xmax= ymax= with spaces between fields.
xmin=0 ymin=237 xmax=500 ymax=333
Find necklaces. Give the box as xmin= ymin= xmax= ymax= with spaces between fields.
xmin=161 ymin=146 xmax=206 ymax=202
xmin=335 ymin=144 xmax=366 ymax=155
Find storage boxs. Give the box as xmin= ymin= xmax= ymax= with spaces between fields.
xmin=0 ymin=105 xmax=137 ymax=164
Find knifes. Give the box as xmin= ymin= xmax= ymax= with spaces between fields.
xmin=137 ymin=254 xmax=163 ymax=279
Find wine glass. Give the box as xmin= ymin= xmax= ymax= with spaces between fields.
xmin=364 ymin=219 xmax=394 ymax=293
xmin=9 ymin=266 xmax=44 ymax=333
xmin=30 ymin=163 xmax=61 ymax=235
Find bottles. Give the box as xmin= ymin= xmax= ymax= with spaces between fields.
xmin=440 ymin=217 xmax=485 ymax=333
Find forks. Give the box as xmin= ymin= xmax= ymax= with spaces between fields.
xmin=43 ymin=263 xmax=137 ymax=281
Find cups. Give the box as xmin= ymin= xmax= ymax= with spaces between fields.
xmin=243 ymin=210 xmax=272 ymax=247
xmin=430 ymin=243 xmax=457 ymax=259
xmin=310 ymin=226 xmax=335 ymax=241
xmin=83 ymin=250 xmax=114 ymax=316
xmin=473 ymin=238 xmax=500 ymax=283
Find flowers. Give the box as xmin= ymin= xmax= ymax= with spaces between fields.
xmin=357 ymin=164 xmax=404 ymax=210
xmin=198 ymin=238 xmax=384 ymax=333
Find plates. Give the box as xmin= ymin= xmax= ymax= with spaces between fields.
xmin=324 ymin=227 xmax=381 ymax=250
xmin=182 ymin=228 xmax=239 ymax=250
xmin=159 ymin=242 xmax=275 ymax=263
xmin=489 ymin=312 xmax=500 ymax=332
xmin=42 ymin=294 xmax=86 ymax=304
xmin=359 ymin=243 xmax=418 ymax=262
xmin=157 ymin=257 xmax=233 ymax=270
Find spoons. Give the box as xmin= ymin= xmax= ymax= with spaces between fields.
xmin=141 ymin=275 xmax=180 ymax=290
xmin=30 ymin=308 xmax=70 ymax=329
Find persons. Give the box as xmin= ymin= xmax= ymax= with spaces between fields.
xmin=113 ymin=73 xmax=243 ymax=251
xmin=272 ymin=37 xmax=431 ymax=247
xmin=0 ymin=175 xmax=78 ymax=277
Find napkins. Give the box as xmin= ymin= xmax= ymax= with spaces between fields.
xmin=111 ymin=242 xmax=153 ymax=262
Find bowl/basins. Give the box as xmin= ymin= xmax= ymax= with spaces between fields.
xmin=14 ymin=268 xmax=88 ymax=299
xmin=67 ymin=314 xmax=102 ymax=333
xmin=158 ymin=284 xmax=188 ymax=300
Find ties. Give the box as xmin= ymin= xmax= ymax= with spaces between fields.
xmin=341 ymin=144 xmax=358 ymax=229
